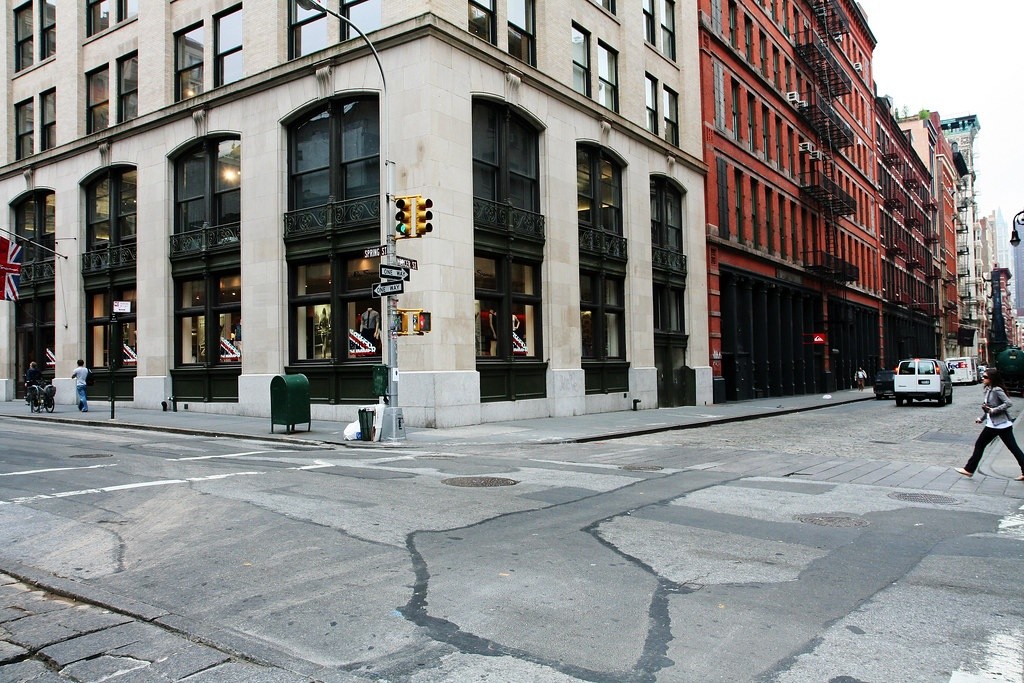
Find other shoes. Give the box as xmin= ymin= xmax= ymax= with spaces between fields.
xmin=954 ymin=467 xmax=973 ymax=477
xmin=1014 ymin=475 xmax=1024 ymax=481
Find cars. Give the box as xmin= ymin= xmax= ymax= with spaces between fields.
xmin=873 ymin=370 xmax=895 ymax=400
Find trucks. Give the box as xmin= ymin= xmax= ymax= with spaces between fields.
xmin=944 ymin=357 xmax=987 ymax=386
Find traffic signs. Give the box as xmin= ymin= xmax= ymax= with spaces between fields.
xmin=379 ymin=264 xmax=410 ymax=281
xmin=372 ymin=280 xmax=404 ymax=299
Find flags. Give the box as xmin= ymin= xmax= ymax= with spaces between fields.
xmin=0 ymin=235 xmax=23 ymax=301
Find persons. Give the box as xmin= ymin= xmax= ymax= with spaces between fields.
xmin=955 ymin=368 xmax=1024 ymax=481
xmin=512 ymin=314 xmax=520 ymax=331
xmin=854 ymin=366 xmax=867 ymax=391
xmin=25 ymin=362 xmax=41 ymax=406
xmin=359 ymin=307 xmax=380 ymax=357
xmin=488 ymin=309 xmax=497 ymax=356
xmin=71 ymin=360 xmax=88 ymax=412
xmin=319 ymin=308 xmax=329 ymax=358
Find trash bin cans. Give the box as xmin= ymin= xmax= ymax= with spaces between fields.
xmin=270 ymin=373 xmax=311 ymax=433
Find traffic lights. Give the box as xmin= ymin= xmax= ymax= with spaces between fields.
xmin=413 ymin=312 xmax=431 ymax=333
xmin=395 ymin=199 xmax=412 ymax=235
xmin=416 ymin=197 xmax=433 ymax=235
xmin=392 ymin=313 xmax=408 ymax=332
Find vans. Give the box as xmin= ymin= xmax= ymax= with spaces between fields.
xmin=893 ymin=358 xmax=955 ymax=406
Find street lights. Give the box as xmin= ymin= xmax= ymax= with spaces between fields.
xmin=295 ymin=0 xmax=407 ymax=442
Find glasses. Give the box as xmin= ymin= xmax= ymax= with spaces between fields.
xmin=983 ymin=376 xmax=989 ymax=379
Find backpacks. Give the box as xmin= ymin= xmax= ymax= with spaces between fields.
xmin=86 ymin=368 xmax=95 ymax=385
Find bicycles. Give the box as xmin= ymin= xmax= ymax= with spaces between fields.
xmin=31 ymin=385 xmax=56 ymax=412
